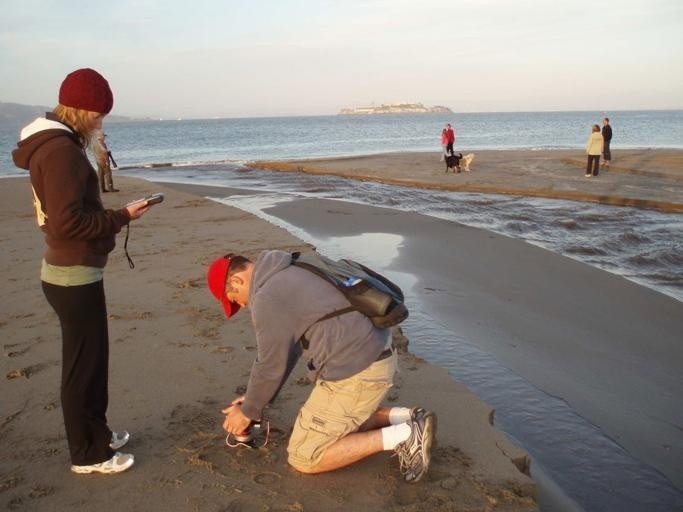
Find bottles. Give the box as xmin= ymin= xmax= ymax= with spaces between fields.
xmin=227 ymin=401 xmax=254 ymax=448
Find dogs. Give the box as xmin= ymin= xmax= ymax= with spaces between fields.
xmin=445 ymin=153 xmax=462 ymax=173
xmin=459 ymin=153 xmax=474 ymax=172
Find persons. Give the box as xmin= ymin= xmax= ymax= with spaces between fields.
xmin=584 ymin=124 xmax=605 ymax=177
xmin=10 ymin=69 xmax=151 ymax=476
xmin=206 ymin=249 xmax=437 ymax=484
xmin=440 ymin=124 xmax=454 ymax=162
xmin=93 ymin=131 xmax=120 ymax=192
xmin=601 ymin=118 xmax=612 ymax=165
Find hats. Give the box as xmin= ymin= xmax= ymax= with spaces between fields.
xmin=58 ymin=68 xmax=113 ymax=114
xmin=207 ymin=253 xmax=242 ymax=319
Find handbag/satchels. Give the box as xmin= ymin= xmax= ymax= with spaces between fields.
xmin=288 ymin=250 xmax=409 ymax=329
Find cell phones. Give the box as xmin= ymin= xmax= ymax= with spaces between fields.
xmin=125 ymin=193 xmax=163 ymax=208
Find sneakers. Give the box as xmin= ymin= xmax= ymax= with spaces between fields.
xmin=102 ymin=189 xmax=120 ymax=193
xmin=394 ymin=405 xmax=436 ymax=484
xmin=69 ymin=430 xmax=135 ymax=475
